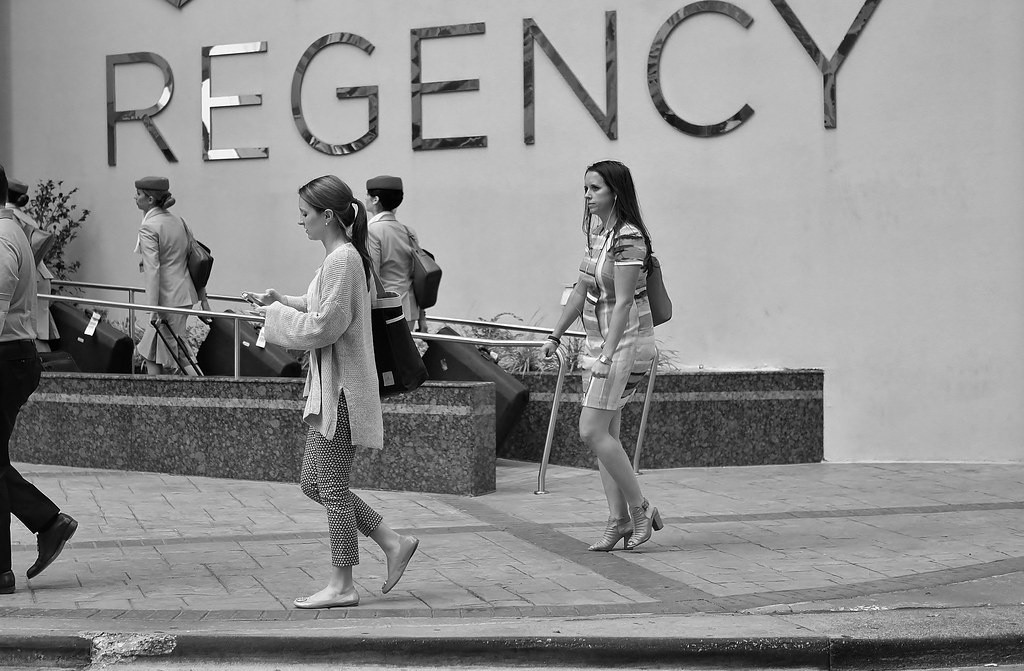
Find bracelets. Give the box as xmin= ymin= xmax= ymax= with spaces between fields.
xmin=548 ymin=335 xmax=560 ymax=347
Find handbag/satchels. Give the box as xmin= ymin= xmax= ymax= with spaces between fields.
xmin=180 ymin=217 xmax=214 ymax=289
xmin=370 ymin=257 xmax=428 ymax=399
xmin=13 ymin=213 xmax=53 ymax=267
xmin=403 ymin=225 xmax=441 ymax=308
xmin=647 ymin=254 xmax=672 ymax=326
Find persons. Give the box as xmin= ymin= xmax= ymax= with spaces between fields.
xmin=540 ymin=161 xmax=664 ymax=552
xmin=0 ymin=165 xmax=78 ymax=594
xmin=131 ymin=176 xmax=213 ymax=376
xmin=361 ymin=175 xmax=428 ymax=336
xmin=241 ymin=176 xmax=419 ymax=611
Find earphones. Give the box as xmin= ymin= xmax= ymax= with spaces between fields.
xmin=615 ymin=194 xmax=617 ymax=199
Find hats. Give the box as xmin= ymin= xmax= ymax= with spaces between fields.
xmin=366 ymin=175 xmax=404 ymax=189
xmin=135 ymin=176 xmax=169 ymax=190
xmin=7 ymin=178 xmax=28 ymax=192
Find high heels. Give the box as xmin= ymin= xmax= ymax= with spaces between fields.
xmin=627 ymin=497 xmax=664 ymax=548
xmin=588 ymin=515 xmax=633 ymax=550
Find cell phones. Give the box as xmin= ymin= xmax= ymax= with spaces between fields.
xmin=244 ymin=293 xmax=266 ymax=306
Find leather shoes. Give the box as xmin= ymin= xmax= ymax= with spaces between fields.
xmin=294 ymin=586 xmax=359 ymax=608
xmin=0 ymin=571 xmax=15 ymax=593
xmin=382 ymin=535 xmax=418 ymax=593
xmin=27 ymin=512 xmax=78 ymax=579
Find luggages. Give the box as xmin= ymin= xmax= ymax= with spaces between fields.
xmin=33 ymin=339 xmax=80 ymax=371
xmin=196 ymin=309 xmax=301 ymax=377
xmin=414 ymin=325 xmax=528 ymax=456
xmin=47 ymin=300 xmax=136 ymax=373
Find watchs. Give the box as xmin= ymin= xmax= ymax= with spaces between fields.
xmin=599 ymin=353 xmax=613 ymax=366
